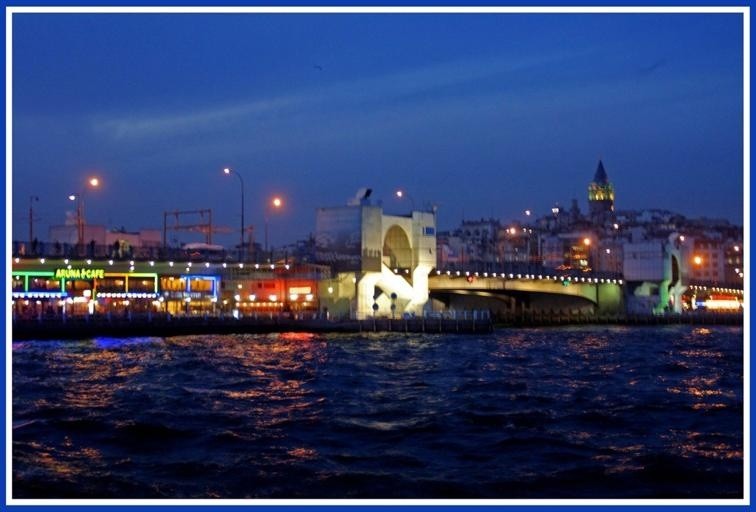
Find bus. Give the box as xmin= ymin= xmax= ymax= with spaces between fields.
xmin=183 ymin=242 xmax=228 ymax=262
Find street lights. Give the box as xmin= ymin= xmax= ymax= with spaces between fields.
xmin=497 ymin=205 xmax=625 ymax=271
xmin=68 ymin=172 xmax=100 ymax=253
xmin=395 ymin=187 xmax=415 ymax=212
xmin=222 ymin=167 xmax=246 ymax=261
xmin=261 ymin=195 xmax=282 ymax=263
